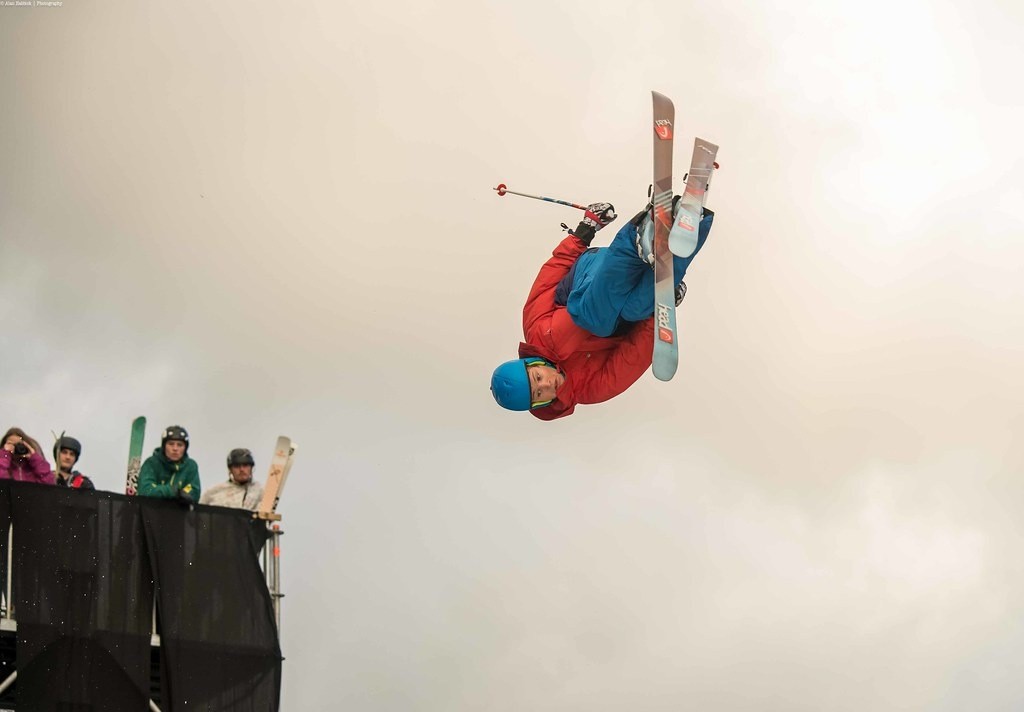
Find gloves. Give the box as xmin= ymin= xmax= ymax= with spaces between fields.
xmin=178 ymin=486 xmax=193 ymax=505
xmin=673 ymin=282 xmax=688 ymax=308
xmin=560 ymin=202 xmax=618 ymax=248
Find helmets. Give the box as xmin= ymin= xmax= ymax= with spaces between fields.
xmin=161 ymin=425 xmax=189 ymax=454
xmin=53 ymin=436 xmax=81 ymax=462
xmin=227 ymin=449 xmax=254 ymax=468
xmin=491 ymin=357 xmax=565 ymax=411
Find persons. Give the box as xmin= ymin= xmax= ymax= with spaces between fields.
xmin=198 ymin=448 xmax=264 ymax=512
xmin=0 ymin=427 xmax=57 ymax=486
xmin=49 ymin=436 xmax=95 ymax=489
xmin=489 ymin=162 xmax=721 ymax=422
xmin=137 ymin=424 xmax=201 ymax=504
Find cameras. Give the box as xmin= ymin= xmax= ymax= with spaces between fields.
xmin=13 ymin=442 xmax=29 ymax=455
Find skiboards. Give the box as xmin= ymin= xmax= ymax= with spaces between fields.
xmin=645 ymin=90 xmax=721 ymax=381
xmin=50 ymin=429 xmax=66 ymax=486
xmin=127 ymin=415 xmax=145 ymax=497
xmin=258 ymin=435 xmax=296 ymax=514
